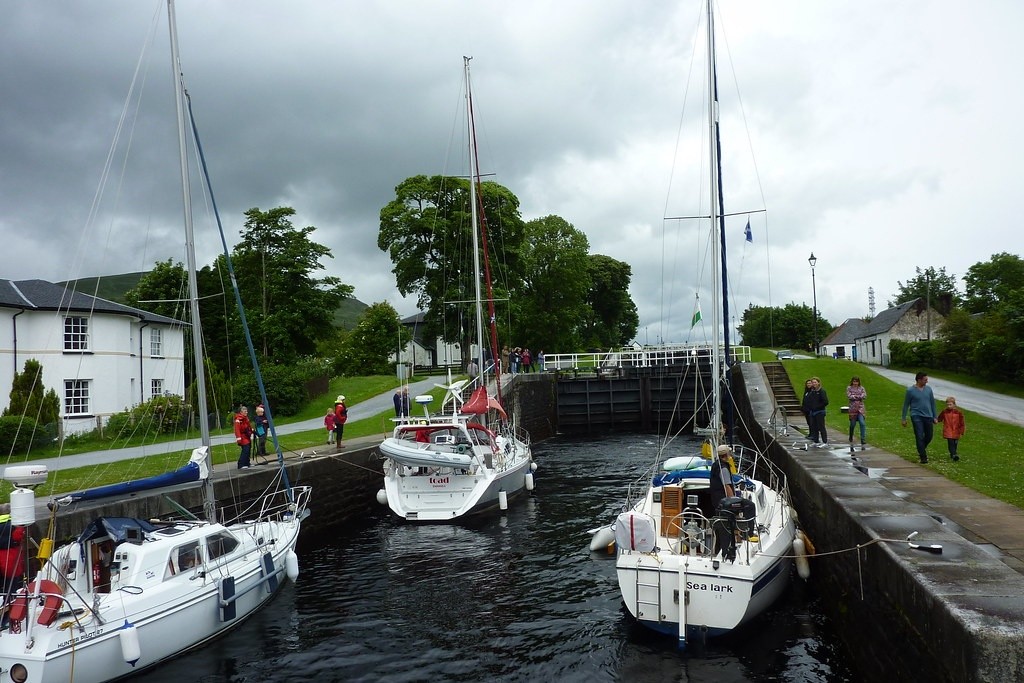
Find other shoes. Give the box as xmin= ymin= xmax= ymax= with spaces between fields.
xmin=816 ymin=443 xmax=828 ymax=449
xmin=806 ymin=436 xmax=815 ymax=440
xmin=327 ymin=441 xmax=330 ymax=445
xmin=849 ymin=436 xmax=853 ymax=442
xmin=921 ymin=458 xmax=927 ymax=463
xmin=332 ymin=441 xmax=335 ymax=444
xmin=249 ymin=465 xmax=255 ymax=467
xmin=808 ymin=442 xmax=821 ymax=447
xmin=242 ymin=466 xmax=248 ymax=469
xmin=257 ymin=453 xmax=270 ymax=456
xmin=861 ymin=439 xmax=866 ymax=444
xmin=950 ymin=454 xmax=959 ymax=461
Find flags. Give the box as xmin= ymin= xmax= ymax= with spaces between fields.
xmin=744 ymin=218 xmax=753 ymax=243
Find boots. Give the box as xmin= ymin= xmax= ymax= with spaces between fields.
xmin=337 ymin=439 xmax=345 ymax=448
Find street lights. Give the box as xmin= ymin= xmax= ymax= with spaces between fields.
xmin=924 ymin=275 xmax=932 ymax=338
xmin=732 ymin=317 xmax=736 ymax=345
xmin=807 ymin=252 xmax=818 ymax=354
xmin=749 ymin=302 xmax=752 ymax=343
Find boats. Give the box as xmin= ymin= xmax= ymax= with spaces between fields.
xmin=589 ymin=521 xmax=615 ymax=553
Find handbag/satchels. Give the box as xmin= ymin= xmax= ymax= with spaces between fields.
xmin=256 ymin=424 xmax=265 ymax=437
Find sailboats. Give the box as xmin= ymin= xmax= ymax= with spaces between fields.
xmin=374 ymin=55 xmax=540 ymax=524
xmin=613 ymin=0 xmax=814 ymax=633
xmin=0 ymin=0 xmax=323 ymax=683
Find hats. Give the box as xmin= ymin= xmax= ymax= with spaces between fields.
xmin=718 ymin=445 xmax=732 ymax=455
xmin=337 ymin=395 xmax=346 ymax=401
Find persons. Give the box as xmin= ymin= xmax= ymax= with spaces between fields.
xmin=801 ymin=377 xmax=830 ymax=449
xmin=392 ymin=386 xmax=413 ymax=425
xmin=323 ymin=408 xmax=336 ymax=445
xmin=485 ymin=341 xmax=536 ymax=376
xmin=846 ymin=376 xmax=868 ymax=443
xmin=537 ymin=350 xmax=546 ymax=373
xmin=234 ymin=406 xmax=253 ymax=469
xmin=709 ymin=444 xmax=737 ymax=516
xmin=467 ymin=359 xmax=479 ymax=382
xmin=901 ymin=372 xmax=937 ymax=464
xmin=937 ymin=396 xmax=965 ymax=461
xmin=334 ymin=394 xmax=347 ymax=448
xmin=253 ymin=407 xmax=271 ymax=456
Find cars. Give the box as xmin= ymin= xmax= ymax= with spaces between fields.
xmin=776 ymin=350 xmax=794 ymax=361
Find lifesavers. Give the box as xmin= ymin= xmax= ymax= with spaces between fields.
xmin=8 ymin=579 xmax=63 ymax=625
